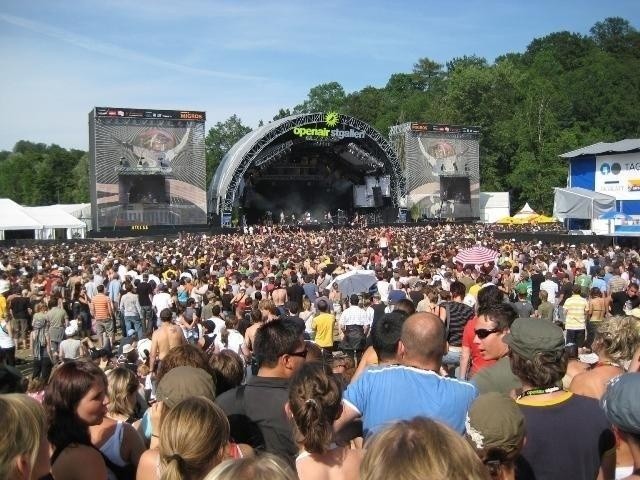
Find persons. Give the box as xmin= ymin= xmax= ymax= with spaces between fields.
xmin=1 ymin=208 xmax=640 ymax=478
xmin=416 ymin=137 xmax=471 ymax=178
xmin=116 ymin=121 xmax=194 ymax=166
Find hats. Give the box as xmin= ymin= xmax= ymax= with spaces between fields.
xmin=122 ymin=344 xmax=135 ymax=353
xmin=464 ymin=392 xmax=526 ymax=454
xmin=502 ymin=317 xmax=565 ymax=362
xmin=64 ymin=326 xmax=76 ymax=336
xmin=199 ymin=320 xmax=215 ymax=332
xmin=127 ymin=329 xmax=137 ymax=337
xmin=598 ymin=372 xmax=640 ymax=434
xmin=155 ymin=366 xmax=218 ymax=410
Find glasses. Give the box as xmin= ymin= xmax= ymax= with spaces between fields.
xmin=475 ymin=329 xmax=501 ymax=339
xmin=288 ymin=345 xmax=308 ymax=359
